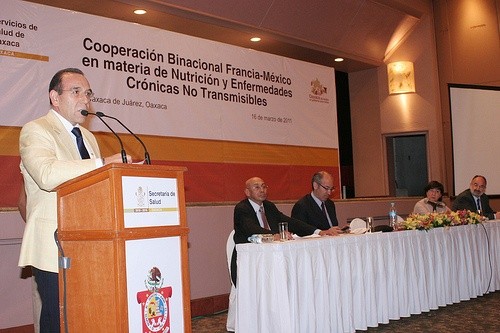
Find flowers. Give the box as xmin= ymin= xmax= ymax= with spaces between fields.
xmin=396 ymin=209 xmax=486 ymax=232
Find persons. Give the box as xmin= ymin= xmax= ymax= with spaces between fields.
xmin=17 ymin=68 xmax=132 ymax=333
xmin=413 ymin=181 xmax=452 ymax=214
xmin=451 ymin=174 xmax=500 ymax=220
xmin=231 ymin=176 xmax=339 ymax=289
xmin=290 ymin=170 xmax=339 ymax=230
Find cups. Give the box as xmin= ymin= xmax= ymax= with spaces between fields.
xmin=279 ymin=222 xmax=288 ymax=240
xmin=477 ymin=209 xmax=483 ymax=217
xmin=367 ymin=216 xmax=374 ymax=230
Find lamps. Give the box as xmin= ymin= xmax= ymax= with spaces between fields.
xmin=387 ymin=61 xmax=415 ymax=95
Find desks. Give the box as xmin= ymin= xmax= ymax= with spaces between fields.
xmin=237 ymin=219 xmax=500 ymax=333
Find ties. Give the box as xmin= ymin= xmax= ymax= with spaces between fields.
xmin=477 ymin=198 xmax=480 ymax=211
xmin=428 ymin=201 xmax=443 ymax=212
xmin=259 ymin=207 xmax=269 ymax=230
xmin=321 ymin=202 xmax=331 ymax=228
xmin=71 ymin=127 xmax=91 ymax=159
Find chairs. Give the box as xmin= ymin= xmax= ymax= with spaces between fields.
xmin=350 ymin=218 xmax=367 ymax=231
xmin=226 ymin=229 xmax=237 ymax=332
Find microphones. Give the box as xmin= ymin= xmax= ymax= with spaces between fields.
xmin=81 ymin=110 xmax=128 ymax=163
xmin=424 ymin=198 xmax=443 ymax=207
xmin=96 ymin=111 xmax=150 ymax=165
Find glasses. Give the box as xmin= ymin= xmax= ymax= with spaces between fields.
xmin=58 ymin=89 xmax=95 ymax=99
xmin=315 ymin=181 xmax=335 ymax=192
xmin=428 ymin=189 xmax=441 ymax=195
xmin=248 ymin=185 xmax=268 ymax=190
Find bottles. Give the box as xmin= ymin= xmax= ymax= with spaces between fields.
xmin=389 ymin=203 xmax=398 ymax=226
